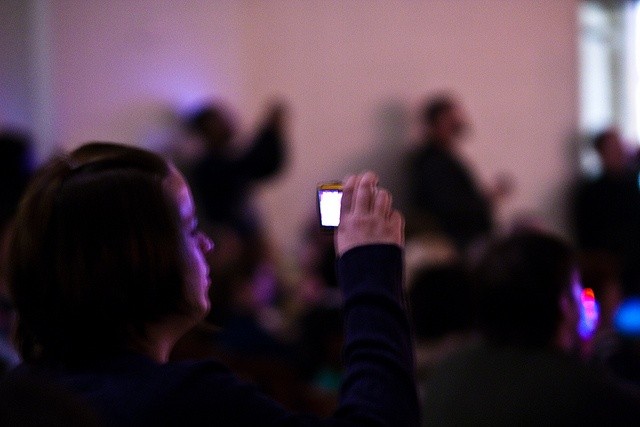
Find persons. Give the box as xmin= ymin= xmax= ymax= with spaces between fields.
xmin=391 ymin=92 xmax=517 ymax=243
xmin=570 ymin=126 xmax=640 ymax=276
xmin=3 ymin=140 xmax=421 ymax=427
xmin=460 ymin=232 xmax=640 ymax=422
xmin=170 ymin=99 xmax=288 ymax=262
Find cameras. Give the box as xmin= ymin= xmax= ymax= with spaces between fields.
xmin=317 ymin=184 xmax=344 ymax=228
